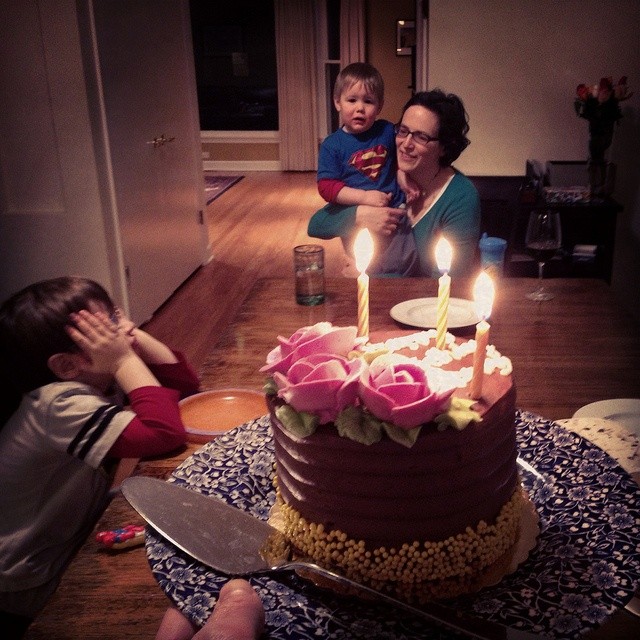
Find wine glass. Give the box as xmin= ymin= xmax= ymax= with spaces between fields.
xmin=523 ymin=210 xmax=563 ymax=302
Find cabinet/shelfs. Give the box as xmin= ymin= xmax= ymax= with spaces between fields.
xmin=465 ymin=176 xmax=624 ymax=286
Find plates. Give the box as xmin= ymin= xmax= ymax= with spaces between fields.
xmin=177 ymin=388 xmax=273 ymax=444
xmin=571 ymin=398 xmax=640 ymax=441
xmin=389 ymin=297 xmax=485 ymax=330
xmin=144 ymin=409 xmax=640 ymax=640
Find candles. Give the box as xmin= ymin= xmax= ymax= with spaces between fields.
xmin=431 ymin=234 xmax=455 ymax=350
xmin=470 ymin=272 xmax=495 ymax=400
xmin=351 ymin=226 xmax=376 ymax=346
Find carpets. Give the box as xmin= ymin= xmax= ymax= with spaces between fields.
xmin=205 ymin=175 xmax=243 ymax=205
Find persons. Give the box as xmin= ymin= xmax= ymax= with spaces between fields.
xmin=315 ymin=62 xmax=420 ymax=278
xmin=307 ymin=85 xmax=480 ymax=280
xmin=1 ymin=275 xmax=203 ymax=620
xmin=153 ymin=577 xmax=267 ymax=639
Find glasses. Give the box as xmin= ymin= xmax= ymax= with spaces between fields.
xmin=394 ymin=123 xmax=445 ymax=146
xmin=102 ymin=306 xmax=123 ymax=332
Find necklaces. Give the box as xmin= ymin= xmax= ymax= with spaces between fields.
xmin=409 ymin=164 xmax=445 ymax=198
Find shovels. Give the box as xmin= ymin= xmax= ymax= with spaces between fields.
xmin=121 ymin=476 xmax=550 ymax=639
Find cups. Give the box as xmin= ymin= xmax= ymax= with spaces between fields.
xmin=478 ymin=232 xmax=507 ymax=276
xmin=293 ymin=245 xmax=326 ymax=306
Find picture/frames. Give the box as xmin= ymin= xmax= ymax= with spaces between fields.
xmin=395 ymin=17 xmax=416 ymax=57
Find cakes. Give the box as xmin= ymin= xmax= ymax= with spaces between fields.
xmin=259 ymin=320 xmax=531 ymax=582
